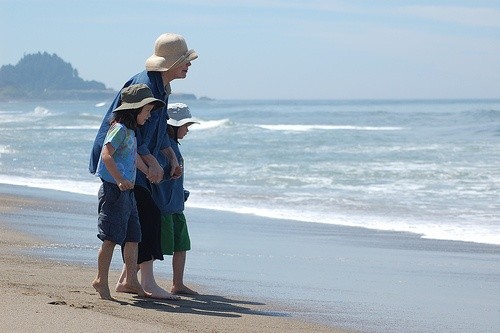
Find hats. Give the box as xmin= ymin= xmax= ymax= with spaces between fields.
xmin=166 ymin=103 xmax=200 ymax=126
xmin=145 ymin=33 xmax=198 ymax=72
xmin=112 ymin=83 xmax=166 ymax=113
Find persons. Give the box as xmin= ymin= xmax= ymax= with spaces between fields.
xmin=89 ymin=33 xmax=198 ymax=299
xmin=92 ymin=83 xmax=160 ymax=299
xmin=115 ymin=103 xmax=200 ymax=296
